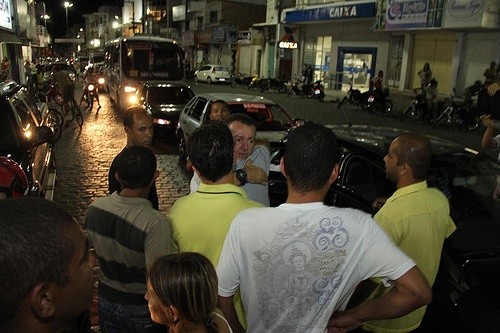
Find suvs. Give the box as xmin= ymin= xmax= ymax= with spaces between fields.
xmin=0 ymin=52 xmax=105 ymax=199
xmin=268 ymin=123 xmax=500 ymax=333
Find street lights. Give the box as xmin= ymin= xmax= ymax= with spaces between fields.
xmin=64 ymin=1 xmax=69 ymax=39
xmin=42 ymin=15 xmax=50 ymax=36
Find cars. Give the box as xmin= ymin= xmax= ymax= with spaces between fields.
xmin=193 ymin=64 xmax=231 ymax=86
xmin=175 ymin=92 xmax=305 ymax=161
xmin=135 ymin=80 xmax=195 ymax=137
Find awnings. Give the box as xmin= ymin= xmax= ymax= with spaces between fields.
xmin=0 ymin=33 xmax=28 ymax=46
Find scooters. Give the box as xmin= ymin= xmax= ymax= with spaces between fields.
xmin=231 ymin=70 xmax=326 ymax=103
xmin=367 ymin=85 xmax=394 ymax=115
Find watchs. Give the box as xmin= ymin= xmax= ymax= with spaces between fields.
xmin=235 ymin=168 xmax=247 ymax=186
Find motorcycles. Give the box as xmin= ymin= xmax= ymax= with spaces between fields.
xmin=398 ymin=88 xmax=437 ymax=125
xmin=337 ymin=78 xmax=373 ymax=111
xmin=432 ymin=87 xmax=482 ymax=132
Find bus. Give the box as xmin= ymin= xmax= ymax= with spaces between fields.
xmin=102 ymin=34 xmax=189 ymax=113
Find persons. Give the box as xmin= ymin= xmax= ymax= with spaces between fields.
xmin=144 ymin=252 xmax=233 ymax=333
xmin=0 ymin=155 xmax=28 ymax=200
xmin=108 ymin=107 xmax=159 ymax=210
xmin=24 ymin=59 xmax=37 ymax=81
xmin=85 ymin=145 xmax=171 ymax=333
xmin=0 ymin=197 xmax=97 ymax=333
xmin=417 ymin=62 xmax=431 ymax=87
xmin=215 ymin=124 xmax=433 ymax=333
xmin=480 ymin=114 xmax=500 ymax=199
xmin=372 ymin=71 xmax=383 ymax=89
xmin=77 ymin=68 xmax=102 ymax=108
xmin=186 ymin=99 xmax=231 ymax=171
xmin=167 ymin=122 xmax=266 ymax=331
xmin=2 ymin=56 xmax=10 ymax=74
xmin=460 ymin=80 xmax=481 ymax=110
xmin=189 ymin=113 xmax=272 ymax=208
xmin=302 ymin=63 xmax=313 ymax=84
xmin=360 ymin=130 xmax=456 ymax=333
xmin=482 ymin=61 xmax=500 ymax=97
